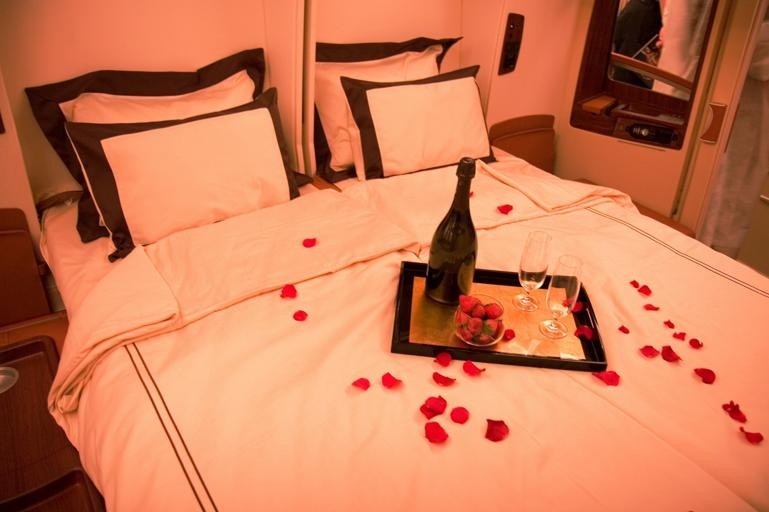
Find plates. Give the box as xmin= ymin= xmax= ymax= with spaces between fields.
xmin=454 ymin=324 xmax=505 ymax=346
xmin=391 ymin=261 xmax=607 ymax=372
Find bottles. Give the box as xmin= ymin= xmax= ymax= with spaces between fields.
xmin=426 ymin=158 xmax=477 ymax=304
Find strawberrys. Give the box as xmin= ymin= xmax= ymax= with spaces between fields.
xmin=456 ymin=294 xmax=503 ymax=345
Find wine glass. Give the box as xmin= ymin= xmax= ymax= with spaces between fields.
xmin=538 ymin=255 xmax=583 ymax=339
xmin=511 ymin=231 xmax=552 ymax=312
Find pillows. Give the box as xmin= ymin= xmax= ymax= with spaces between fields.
xmin=63 ymin=86 xmax=301 ymax=263
xmin=339 ymin=65 xmax=496 ymax=182
xmin=24 ymin=48 xmax=315 ymax=244
xmin=316 ymin=37 xmax=466 ymax=184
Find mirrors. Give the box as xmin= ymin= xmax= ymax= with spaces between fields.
xmin=606 ymin=0 xmax=715 ymax=103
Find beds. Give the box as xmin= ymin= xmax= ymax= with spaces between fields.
xmin=36 ymin=145 xmax=769 ymax=512
